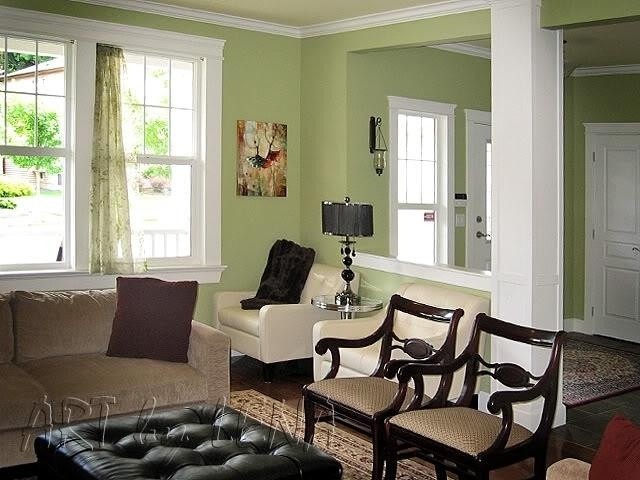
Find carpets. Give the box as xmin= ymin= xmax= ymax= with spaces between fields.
xmin=223 ymin=390 xmax=448 ymax=480
xmin=562 ymin=330 xmax=640 ymax=406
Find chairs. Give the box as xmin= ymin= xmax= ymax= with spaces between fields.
xmin=213 ymin=262 xmax=361 ymax=385
xmin=546 ymin=457 xmax=591 ymax=479
xmin=382 ymin=313 xmax=567 ymax=479
xmin=311 ymin=284 xmax=491 ymax=402
xmin=300 ymin=295 xmax=464 ymax=480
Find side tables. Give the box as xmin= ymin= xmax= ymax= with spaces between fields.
xmin=311 ymin=297 xmax=385 ymax=321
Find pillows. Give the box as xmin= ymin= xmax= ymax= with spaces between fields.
xmin=106 ymin=277 xmax=199 ymax=362
xmin=589 ymin=412 xmax=639 ymax=479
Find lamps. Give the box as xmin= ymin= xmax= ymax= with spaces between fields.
xmin=321 ymin=197 xmax=374 ymax=304
xmin=369 ymin=116 xmax=387 ymax=178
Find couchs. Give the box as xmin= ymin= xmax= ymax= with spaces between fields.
xmin=0 ymin=289 xmax=232 ymax=470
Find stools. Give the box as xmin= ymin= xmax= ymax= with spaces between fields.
xmin=34 ymin=401 xmax=343 ymax=480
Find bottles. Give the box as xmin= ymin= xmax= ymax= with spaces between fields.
xmin=134 ymin=249 xmax=147 ymax=273
